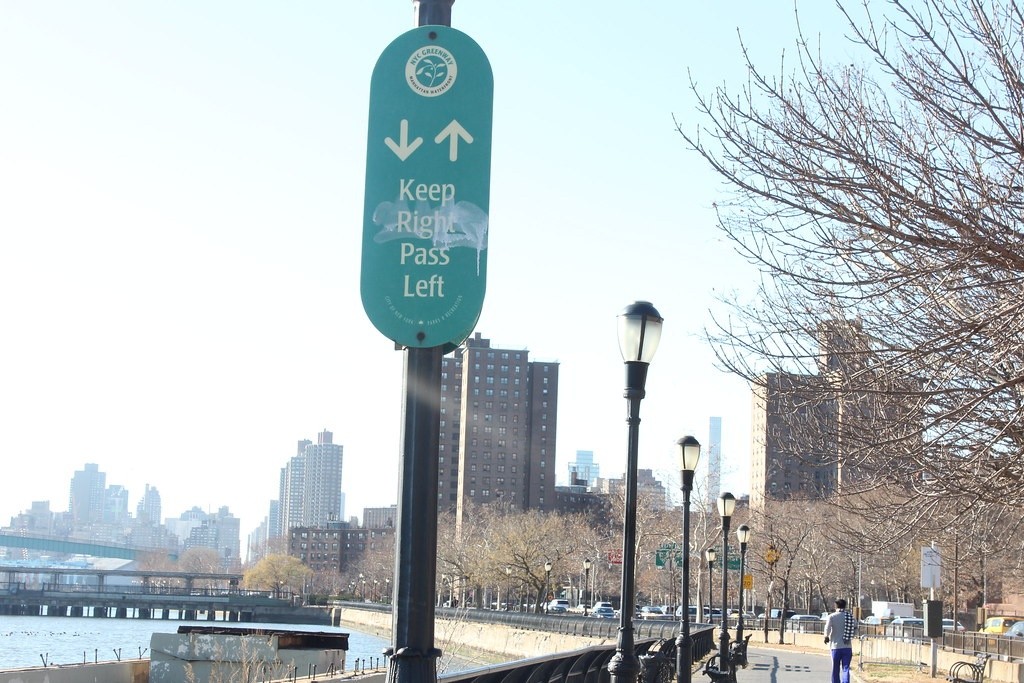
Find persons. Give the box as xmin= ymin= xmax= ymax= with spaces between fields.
xmin=823 ymin=598 xmax=859 ymax=683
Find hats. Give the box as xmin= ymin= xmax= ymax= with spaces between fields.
xmin=835 ymin=600 xmax=845 ymax=608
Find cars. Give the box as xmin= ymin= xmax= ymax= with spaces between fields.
xmin=441 ymin=600 xmax=1024 ymax=650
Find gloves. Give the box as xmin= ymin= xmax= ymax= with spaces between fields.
xmin=825 ymin=637 xmax=829 ymax=644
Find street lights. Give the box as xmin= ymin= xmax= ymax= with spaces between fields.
xmin=672 ymin=435 xmax=700 ymax=682
xmin=582 ymin=558 xmax=592 ymax=616
xmin=506 ymin=565 xmax=512 ymax=612
xmin=715 ymin=491 xmax=737 ymax=672
xmin=544 ymin=561 xmax=551 ymax=616
xmin=373 ymin=579 xmax=378 ymax=604
xmin=608 ymin=298 xmax=665 ymax=683
xmin=704 ymin=548 xmax=715 ymax=625
xmin=736 ymin=524 xmax=750 ymax=645
xmin=362 ymin=578 xmax=366 ymax=602
xmin=385 ymin=577 xmax=390 ymax=604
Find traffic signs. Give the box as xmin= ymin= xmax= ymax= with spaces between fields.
xmin=358 ymin=25 xmax=495 ymax=354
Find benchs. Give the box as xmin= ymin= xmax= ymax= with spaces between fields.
xmin=728 ymin=634 xmax=752 ymax=669
xmin=702 ymin=645 xmax=742 ymax=683
xmin=946 ymin=653 xmax=991 ymax=683
